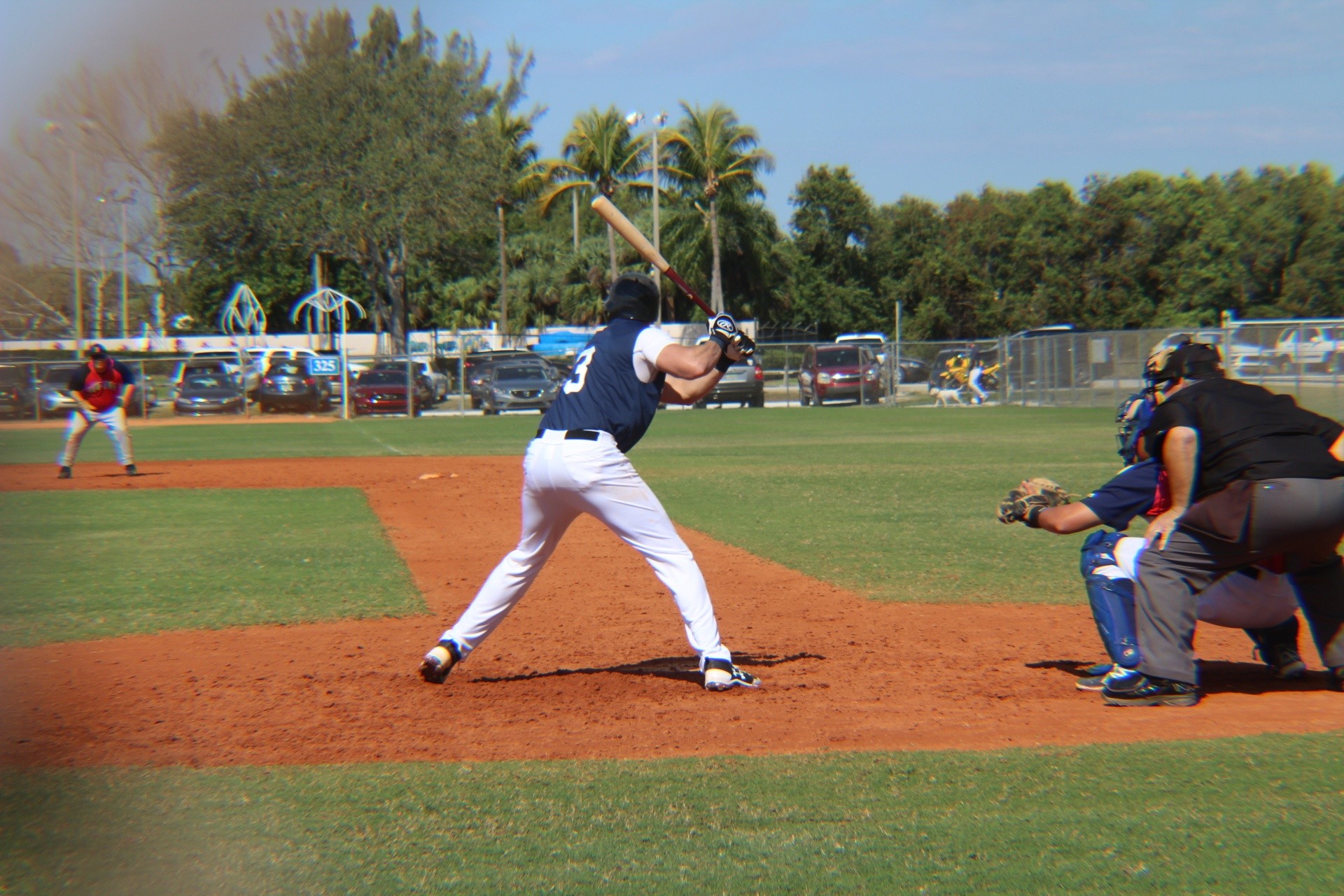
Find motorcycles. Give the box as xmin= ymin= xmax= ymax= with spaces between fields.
xmin=937 ymin=352 xmax=1007 ymax=392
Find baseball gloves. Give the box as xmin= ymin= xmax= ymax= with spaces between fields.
xmin=995 ymin=476 xmax=1073 ymax=525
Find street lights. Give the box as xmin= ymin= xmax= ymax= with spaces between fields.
xmin=624 ymin=109 xmax=670 ymax=324
xmin=44 ymin=121 xmax=85 ymax=341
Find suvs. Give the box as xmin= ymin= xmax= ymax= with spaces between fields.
xmin=692 ymin=335 xmax=765 ymax=410
xmin=188 ymin=348 xmax=260 ymax=401
xmin=370 ymin=360 xmax=433 ymax=412
xmin=983 ymin=324 xmax=1095 ymax=388
xmin=392 ymin=356 xmax=448 ymax=402
xmin=792 ymin=343 xmax=880 ymax=408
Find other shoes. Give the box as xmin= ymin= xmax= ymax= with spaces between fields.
xmin=59 ymin=466 xmax=70 ymax=478
xmin=126 ymin=464 xmax=136 ymax=475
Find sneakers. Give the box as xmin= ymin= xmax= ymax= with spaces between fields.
xmin=420 ymin=640 xmax=461 ymax=685
xmin=1102 ymin=672 xmax=1197 ymax=708
xmin=1076 ymin=663 xmax=1137 ymax=690
xmin=703 ymin=657 xmax=762 ymax=691
xmin=1253 ymin=643 xmax=1305 ymax=679
xmin=1331 ymin=665 xmax=1344 ymax=690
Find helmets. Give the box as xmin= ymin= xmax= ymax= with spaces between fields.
xmin=605 ymin=273 xmax=659 ymax=323
xmin=1141 ymin=332 xmax=1220 ymax=379
xmin=89 ymin=344 xmax=107 ymax=356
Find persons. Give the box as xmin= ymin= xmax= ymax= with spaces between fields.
xmin=996 ymin=332 xmax=1344 ymax=707
xmin=962 ymin=343 xmax=988 ymax=404
xmin=57 ymin=344 xmax=137 ymax=478
xmin=420 ymin=273 xmax=762 ymax=691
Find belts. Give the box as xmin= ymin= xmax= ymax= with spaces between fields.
xmin=1237 ymin=567 xmax=1262 ymax=580
xmin=536 ymin=429 xmax=599 ymax=440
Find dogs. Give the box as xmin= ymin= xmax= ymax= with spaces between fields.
xmin=929 ymin=382 xmax=965 ymax=408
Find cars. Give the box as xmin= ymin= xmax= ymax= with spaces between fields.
xmin=482 ymin=363 xmax=560 ymax=416
xmin=34 ymin=365 xmax=86 ymax=418
xmin=121 ymin=364 xmax=158 ymax=418
xmin=891 ymin=355 xmax=929 ymax=384
xmin=258 ymin=360 xmax=331 ymax=415
xmin=173 ymin=373 xmax=244 ymax=416
xmin=464 ymin=350 xmax=552 ymax=410
xmin=174 ymin=359 xmax=242 ymax=391
xmin=0 ymin=356 xmax=41 ymax=419
xmin=348 ymin=370 xmax=425 ymax=417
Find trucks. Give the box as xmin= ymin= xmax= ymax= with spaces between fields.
xmin=1195 ymin=329 xmax=1273 ymax=376
xmin=1273 ymin=324 xmax=1344 ymax=375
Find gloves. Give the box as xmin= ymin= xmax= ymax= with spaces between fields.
xmin=706 ymin=313 xmax=738 ymax=353
xmin=714 ymin=331 xmax=755 ymax=373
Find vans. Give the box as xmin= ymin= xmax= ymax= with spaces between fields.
xmin=834 ymin=332 xmax=900 ymax=399
xmin=246 ymin=347 xmax=326 ymax=381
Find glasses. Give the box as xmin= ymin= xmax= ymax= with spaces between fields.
xmin=92 ymin=354 xmax=106 ymax=359
xmin=1145 ymin=379 xmax=1175 ymax=392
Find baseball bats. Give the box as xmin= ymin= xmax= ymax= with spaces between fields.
xmin=591 ymin=194 xmax=756 ymax=358
